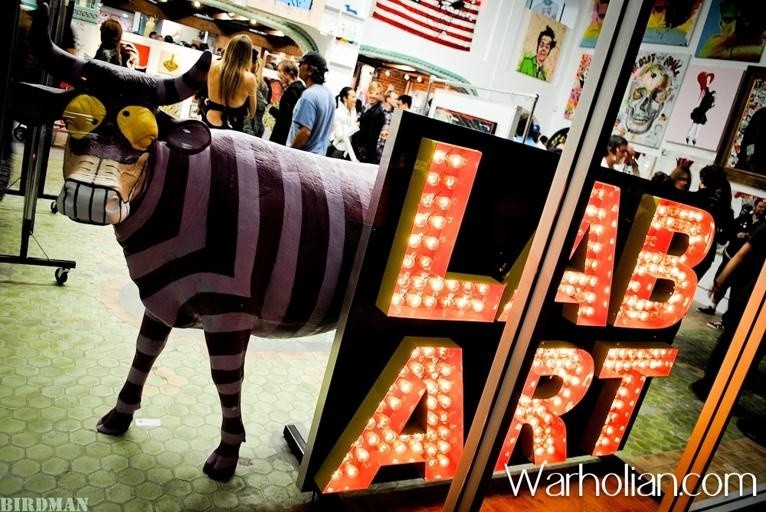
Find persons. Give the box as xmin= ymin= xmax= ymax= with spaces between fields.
xmin=698 ymin=0 xmax=766 ymax=61
xmin=625 ymin=63 xmax=671 ymax=134
xmin=642 ymin=0 xmax=687 ymax=47
xmin=513 ymin=116 xmax=766 ymax=414
xmin=520 ymin=26 xmax=557 ymax=82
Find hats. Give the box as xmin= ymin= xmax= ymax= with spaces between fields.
xmin=295 ymin=51 xmax=329 ymax=73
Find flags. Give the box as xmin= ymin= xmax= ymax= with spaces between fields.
xmin=372 ymin=0 xmax=481 ymax=53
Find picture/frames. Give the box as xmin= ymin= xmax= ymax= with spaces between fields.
xmin=713 ymin=65 xmax=766 ymax=192
xmin=433 ymin=106 xmax=497 ymax=134
